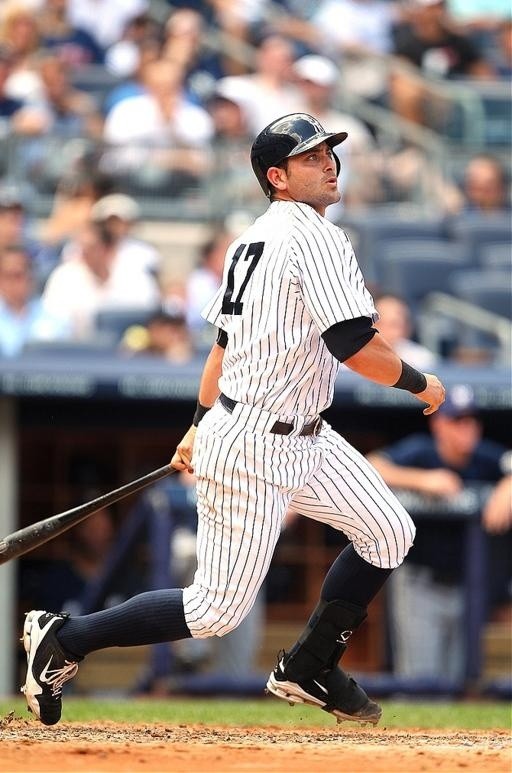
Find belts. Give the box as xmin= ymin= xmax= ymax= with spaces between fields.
xmin=219 ymin=393 xmax=323 ymax=437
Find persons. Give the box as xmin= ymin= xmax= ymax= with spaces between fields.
xmin=62 ymin=450 xmax=262 ymax=699
xmin=363 ymin=380 xmax=512 ymax=700
xmin=19 ymin=109 xmax=449 ymax=726
xmin=41 ymin=508 xmax=113 ymax=578
xmin=0 ymin=0 xmax=510 ymax=371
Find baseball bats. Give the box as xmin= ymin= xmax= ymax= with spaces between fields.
xmin=0 ymin=463 xmax=178 ymax=563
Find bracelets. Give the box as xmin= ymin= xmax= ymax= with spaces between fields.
xmin=390 ymin=357 xmax=428 ymax=395
xmin=192 ymin=398 xmax=211 ymax=427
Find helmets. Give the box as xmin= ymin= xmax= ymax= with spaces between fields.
xmin=250 ymin=113 xmax=349 ymax=176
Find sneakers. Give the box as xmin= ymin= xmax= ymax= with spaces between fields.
xmin=18 ymin=610 xmax=85 ymax=725
xmin=266 ymin=648 xmax=383 ymax=726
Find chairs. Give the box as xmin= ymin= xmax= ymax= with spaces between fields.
xmin=339 ymin=208 xmax=511 ymax=347
